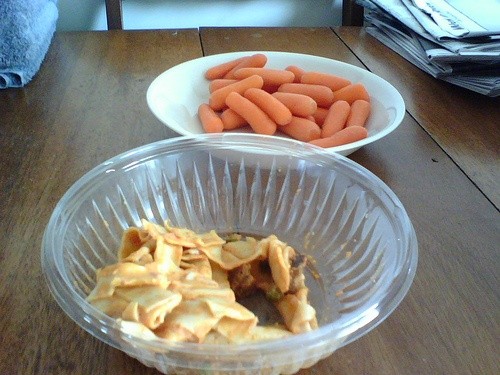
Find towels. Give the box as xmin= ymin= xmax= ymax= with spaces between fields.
xmin=0 ymin=0 xmax=59 ymax=90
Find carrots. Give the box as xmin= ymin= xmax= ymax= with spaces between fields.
xmin=198 ymin=52 xmax=370 ymax=149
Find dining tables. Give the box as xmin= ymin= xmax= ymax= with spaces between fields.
xmin=0 ymin=26 xmax=500 ymax=375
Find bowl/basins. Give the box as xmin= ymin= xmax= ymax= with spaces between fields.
xmin=147 ymin=50 xmax=406 ymax=169
xmin=40 ymin=132 xmax=419 ymax=375
